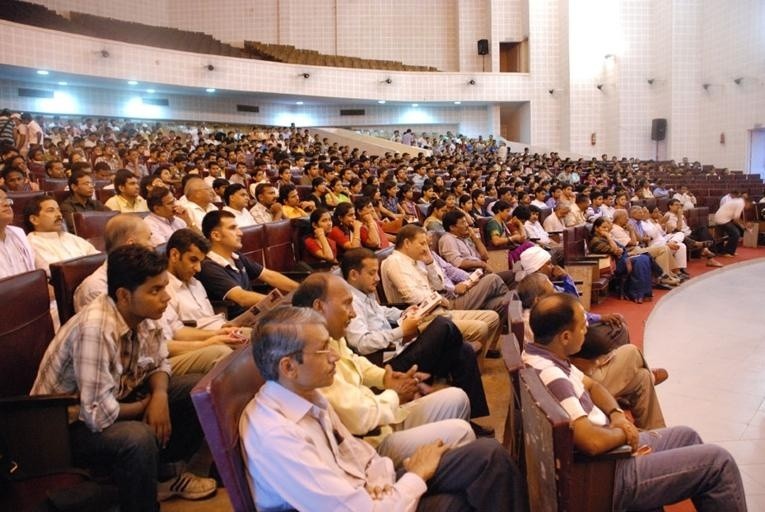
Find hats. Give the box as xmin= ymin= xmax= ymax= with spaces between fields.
xmin=518 ymin=244 xmax=553 ymax=276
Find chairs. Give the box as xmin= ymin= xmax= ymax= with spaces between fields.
xmin=235 ymin=226 xmax=267 ymax=272
xmin=290 ymin=217 xmax=328 ymax=272
xmin=477 ymin=216 xmax=513 ymax=275
xmin=517 ymin=366 xmax=632 ymax=511
xmin=0 ymin=269 xmax=83 ymax=481
xmin=497 ymin=333 xmax=525 ymax=444
xmin=265 ymin=222 xmax=296 ymax=274
xmin=415 ymin=204 xmax=429 ymax=227
xmin=681 ymin=202 xmax=765 ymax=262
xmin=374 ymin=246 xmax=411 ymax=309
xmin=74 ymin=211 xmax=120 ymax=247
xmin=506 ymin=298 xmax=525 ymax=354
xmin=189 ymin=343 xmax=268 ymax=512
xmin=10 ymin=190 xmax=56 ymax=232
xmin=539 ymin=207 xmax=554 ymax=224
xmin=48 ymin=252 xmax=113 ymax=325
xmin=496 ymin=333 xmax=525 ymax=408
xmin=0 ymin=0 xmax=445 ymax=72
xmin=574 ymin=225 xmax=610 ymax=279
xmin=0 ymin=108 xmax=765 ymax=204
xmin=564 ymin=227 xmax=607 ymax=306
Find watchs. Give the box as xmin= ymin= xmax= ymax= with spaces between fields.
xmin=186 ymin=222 xmax=195 ymax=229
xmin=606 ymin=407 xmax=626 ymax=416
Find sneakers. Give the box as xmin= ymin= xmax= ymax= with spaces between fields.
xmin=155 ymin=470 xmax=218 ymax=502
xmin=660 ymin=274 xmax=681 ymax=284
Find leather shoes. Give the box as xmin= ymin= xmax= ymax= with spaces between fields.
xmin=649 ymin=367 xmax=668 ymax=386
xmin=672 ymin=274 xmax=684 ymax=282
xmin=678 ymin=272 xmax=690 ymax=279
xmin=706 ymin=260 xmax=723 ymax=267
xmin=468 ymin=418 xmax=497 ymax=440
xmin=681 ymin=267 xmax=690 ymax=275
xmin=654 ymin=282 xmax=674 ymax=290
xmin=701 ymin=240 xmax=713 ymax=247
xmin=702 ymin=249 xmax=716 ymax=257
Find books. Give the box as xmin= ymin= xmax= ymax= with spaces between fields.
xmin=217 ymin=287 xmax=288 ymax=328
xmin=391 ymin=290 xmax=447 ymax=334
xmin=450 ymin=267 xmax=486 ymax=293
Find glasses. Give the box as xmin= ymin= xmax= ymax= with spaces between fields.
xmin=0 ymin=199 xmax=18 ymax=210
xmin=278 ymin=336 xmax=332 ymax=360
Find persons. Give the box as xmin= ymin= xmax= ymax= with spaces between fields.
xmin=276 ymin=182 xmax=314 ymax=219
xmin=383 ymin=152 xmax=508 ymax=183
xmin=275 ymin=159 xmax=297 ymax=190
xmin=542 ymin=202 xmax=569 ymax=234
xmin=322 ymin=167 xmax=338 ymax=183
xmin=512 ymin=192 xmax=542 ymax=220
xmin=29 ymin=243 xmax=218 ymax=512
xmin=442 ymin=192 xmax=457 ymax=212
xmin=487 ymin=186 xmax=519 ymax=217
xmin=340 ymin=169 xmax=353 ymax=185
xmin=396 ymin=183 xmax=420 ymax=224
xmin=203 ymin=161 xmax=225 ymax=189
xmin=0 ymin=108 xmax=18 ymax=153
xmin=675 ymin=156 xmax=736 ymax=179
xmin=216 ymin=155 xmax=228 ymax=173
xmin=8 ymin=112 xmax=30 ymax=160
xmin=155 ymin=167 xmax=174 ymax=189
xmin=484 ymin=200 xmax=528 ymax=249
xmin=368 ymin=175 xmax=380 ymax=186
xmin=304 ymin=208 xmax=338 ymax=264
xmin=325 ymin=177 xmax=352 ymax=207
xmin=520 ymin=292 xmax=749 ymax=512
xmin=467 ymin=183 xmax=480 ymax=195
xmin=600 ymin=193 xmax=616 ymax=218
xmin=197 ymin=122 xmax=382 ymax=159
xmin=518 ymin=243 xmax=630 ymax=347
xmin=58 ymin=170 xmax=112 ymax=235
xmin=45 ymin=159 xmax=67 ymax=179
xmin=456 ymin=195 xmax=475 ymax=217
xmin=411 ymin=221 xmax=511 ymax=332
xmin=608 ymin=208 xmax=678 ymax=290
xmin=626 ymin=205 xmax=681 ymax=286
xmin=27 ymin=147 xmax=46 ymax=162
xmin=604 ymin=217 xmax=680 ymax=299
xmin=228 ymin=161 xmax=253 ymax=187
xmin=329 ymin=202 xmax=362 ymax=249
xmin=176 ymin=178 xmax=221 ymax=235
xmin=120 ymin=119 xmax=197 ymax=156
xmin=614 ymin=194 xmax=628 ymax=211
xmin=0 ymin=145 xmax=29 ymax=171
xmin=348 ymin=177 xmax=368 ymax=202
xmin=251 ymin=160 xmax=275 ymax=182
xmin=221 ymin=184 xmax=257 ymax=228
xmin=416 ymin=184 xmax=440 ymax=218
xmin=507 ymin=147 xmax=674 ymax=199
xmin=639 ymin=205 xmax=689 ymax=281
xmin=354 ymin=197 xmax=392 ymax=250
xmin=291 ymin=271 xmax=478 ymax=461
xmin=486 ymin=184 xmax=498 ymax=198
xmin=89 ymin=162 xmax=118 ymax=186
xmin=713 ymin=190 xmax=753 ymax=257
xmin=362 ymin=186 xmax=402 ymax=228
xmin=302 ymin=162 xmax=322 ymax=184
xmin=249 ymin=182 xmax=285 ymax=225
xmin=360 ymin=159 xmax=382 ymax=177
xmin=304 ymin=177 xmax=336 ymax=212
xmin=290 ymin=155 xmax=306 ymax=177
xmin=564 ymin=193 xmax=589 ymax=227
xmin=187 ymin=158 xmax=211 ymax=178
xmin=141 ymin=184 xmax=205 ymax=250
xmin=402 ymin=128 xmax=510 ymax=151
xmin=104 ymin=168 xmax=151 ymax=212
xmin=451 ymin=182 xmax=470 ymax=195
xmin=380 ymin=221 xmax=502 ymax=379
xmin=150 ymin=151 xmax=170 ymax=174
xmin=123 ymin=149 xmax=149 ymax=177
xmin=140 ymin=174 xmax=167 ymax=198
xmin=515 ymin=271 xmax=669 ymax=390
xmin=418 ymin=199 xmax=449 ymax=236
xmin=379 ymin=180 xmax=407 ymax=220
xmin=72 ymin=212 xmax=254 ymax=376
xmin=211 ymin=179 xmax=230 ymax=202
xmin=438 ymin=209 xmax=516 ymax=289
xmin=662 ymin=199 xmax=724 ymax=267
xmin=3 ymin=168 xmax=34 ymax=193
xmin=584 ymin=191 xmax=609 ymax=222
xmin=525 ymin=204 xmax=556 ymax=251
xmin=168 ymin=154 xmax=188 ymax=179
xmin=351 ymin=161 xmax=360 ymax=176
xmin=391 ymin=129 xmax=401 ymax=143
xmin=235 ymin=303 xmax=519 ymax=511
xmin=45 ymin=115 xmax=121 ymax=160
xmin=20 ymin=112 xmax=45 ymax=158
xmin=587 ymin=217 xmax=654 ymax=303
xmin=470 ymin=189 xmax=495 ymax=221
xmin=199 ymin=209 xmax=300 ymax=312
xmin=507 ymin=206 xmax=540 ymax=244
xmin=671 ymin=184 xmax=698 ymax=210
xmin=0 ymin=188 xmax=61 ymax=332
xmin=23 ymin=194 xmax=102 ymax=279
xmin=152 ymin=228 xmax=253 ymax=338
xmin=333 ymin=160 xmax=345 ymax=174
xmin=640 ymin=205 xmax=713 ymax=282
xmin=336 ymin=246 xmax=496 ymax=437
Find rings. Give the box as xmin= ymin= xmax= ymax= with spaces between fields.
xmin=414 ymin=378 xmax=419 ymax=383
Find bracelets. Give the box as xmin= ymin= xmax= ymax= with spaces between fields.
xmin=507 ymin=236 xmax=514 ymax=243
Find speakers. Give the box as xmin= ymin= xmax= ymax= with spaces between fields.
xmin=651 ymin=119 xmax=666 ymax=140
xmin=478 ymin=39 xmax=488 ymax=55
